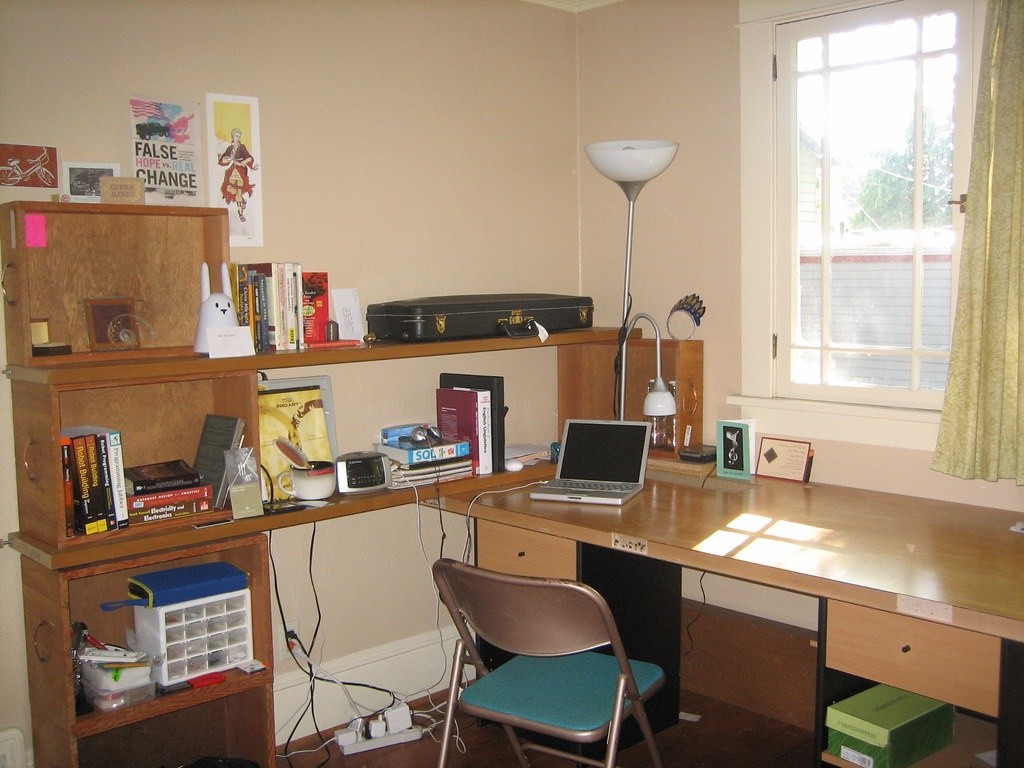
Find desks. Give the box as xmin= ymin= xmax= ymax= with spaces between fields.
xmin=417 ymin=454 xmax=1024 ymax=768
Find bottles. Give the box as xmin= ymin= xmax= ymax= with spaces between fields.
xmin=645 ymin=378 xmax=666 ymax=447
xmin=664 ymin=381 xmax=677 ymax=448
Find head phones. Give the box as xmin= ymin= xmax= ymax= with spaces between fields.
xmin=411 ymin=426 xmax=443 ymax=442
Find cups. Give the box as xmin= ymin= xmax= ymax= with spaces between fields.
xmin=277 ymin=460 xmax=336 ymax=501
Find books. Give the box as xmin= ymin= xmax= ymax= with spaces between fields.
xmin=238 ymin=263 xmax=360 ymax=353
xmin=61 ymin=414 xmax=245 ymax=539
xmin=373 ymin=373 xmax=504 ymax=489
xmin=80 ymin=648 xmax=147 ymax=663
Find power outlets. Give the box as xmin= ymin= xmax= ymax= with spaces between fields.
xmin=280 ymin=622 xmax=301 ymax=661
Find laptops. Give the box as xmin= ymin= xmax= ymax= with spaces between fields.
xmin=529 ymin=418 xmax=652 ymax=506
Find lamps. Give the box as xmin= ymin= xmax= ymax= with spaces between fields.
xmin=585 ymin=138 xmax=677 ymax=421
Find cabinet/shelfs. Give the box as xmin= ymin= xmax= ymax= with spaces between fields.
xmin=0 ymin=338 xmax=714 ymax=768
xmin=134 ymin=588 xmax=256 ymax=686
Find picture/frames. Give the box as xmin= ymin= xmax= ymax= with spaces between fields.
xmin=84 ymin=295 xmax=141 ymax=349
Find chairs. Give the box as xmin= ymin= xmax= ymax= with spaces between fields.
xmin=432 ymin=559 xmax=664 ymax=768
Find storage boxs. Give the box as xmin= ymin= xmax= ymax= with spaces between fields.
xmin=1 ymin=199 xmax=230 ymax=367
xmin=826 ymin=685 xmax=956 ymax=768
xmin=81 ymin=680 xmax=157 ymax=712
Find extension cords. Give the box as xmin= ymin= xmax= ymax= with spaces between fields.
xmin=339 ymin=725 xmax=423 ymax=755
